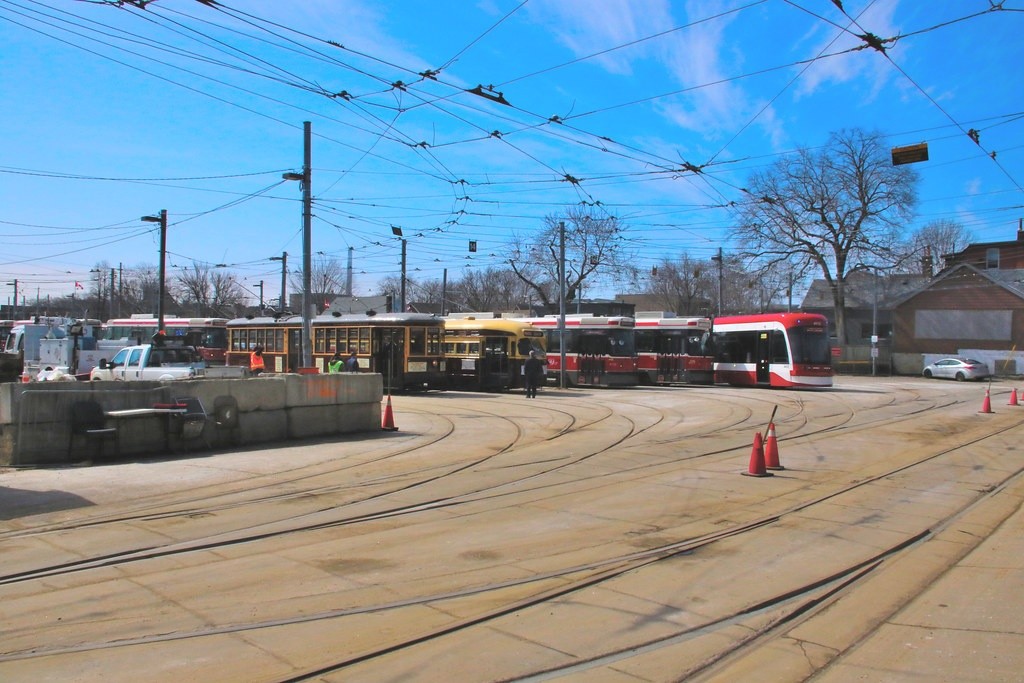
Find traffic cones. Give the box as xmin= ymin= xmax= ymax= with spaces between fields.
xmin=381 ymin=394 xmax=400 ymax=431
xmin=763 ymin=423 xmax=785 ymax=470
xmin=978 ymin=391 xmax=997 ymax=413
xmin=1020 ymin=391 xmax=1024 ymax=401
xmin=1006 ymin=388 xmax=1022 ymax=405
xmin=740 ymin=431 xmax=775 ymax=477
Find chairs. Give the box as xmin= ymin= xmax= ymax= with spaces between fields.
xmin=175 ymin=395 xmax=221 ymax=455
xmin=204 ymin=397 xmax=240 ymax=448
xmin=63 ymin=399 xmax=120 ymax=464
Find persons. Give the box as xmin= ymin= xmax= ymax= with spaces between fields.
xmin=345 ymin=353 xmax=360 ymax=373
xmin=250 ymin=345 xmax=264 ymax=377
xmin=524 ymin=351 xmax=538 ymax=399
xmin=328 ymin=353 xmax=345 ymax=374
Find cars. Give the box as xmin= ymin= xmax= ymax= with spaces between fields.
xmin=922 ymin=358 xmax=990 ymax=382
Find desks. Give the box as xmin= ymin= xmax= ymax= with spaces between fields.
xmin=106 ymin=408 xmax=190 ymax=455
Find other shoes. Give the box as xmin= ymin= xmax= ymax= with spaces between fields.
xmin=531 ymin=395 xmax=535 ymax=398
xmin=525 ymin=395 xmax=530 ymax=398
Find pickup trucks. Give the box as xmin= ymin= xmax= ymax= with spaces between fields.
xmin=90 ymin=341 xmax=251 ymax=381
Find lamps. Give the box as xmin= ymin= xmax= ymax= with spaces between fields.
xmin=890 ymin=141 xmax=931 ymax=166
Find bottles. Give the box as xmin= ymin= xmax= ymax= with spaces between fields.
xmin=22 ymin=368 xmax=29 ymax=382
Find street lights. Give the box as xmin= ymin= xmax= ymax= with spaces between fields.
xmin=7 ymin=279 xmax=18 ymax=320
xmin=280 ymin=171 xmax=313 ymax=368
xmin=390 ymin=226 xmax=408 ymax=312
xmin=253 ymin=279 xmax=264 ymax=315
xmin=141 ymin=209 xmax=167 ymax=335
xmin=269 ymin=252 xmax=288 ymax=310
xmin=710 ymin=246 xmax=724 ymax=314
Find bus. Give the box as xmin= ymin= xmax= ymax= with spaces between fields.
xmin=0 ymin=310 xmax=837 ymax=394
